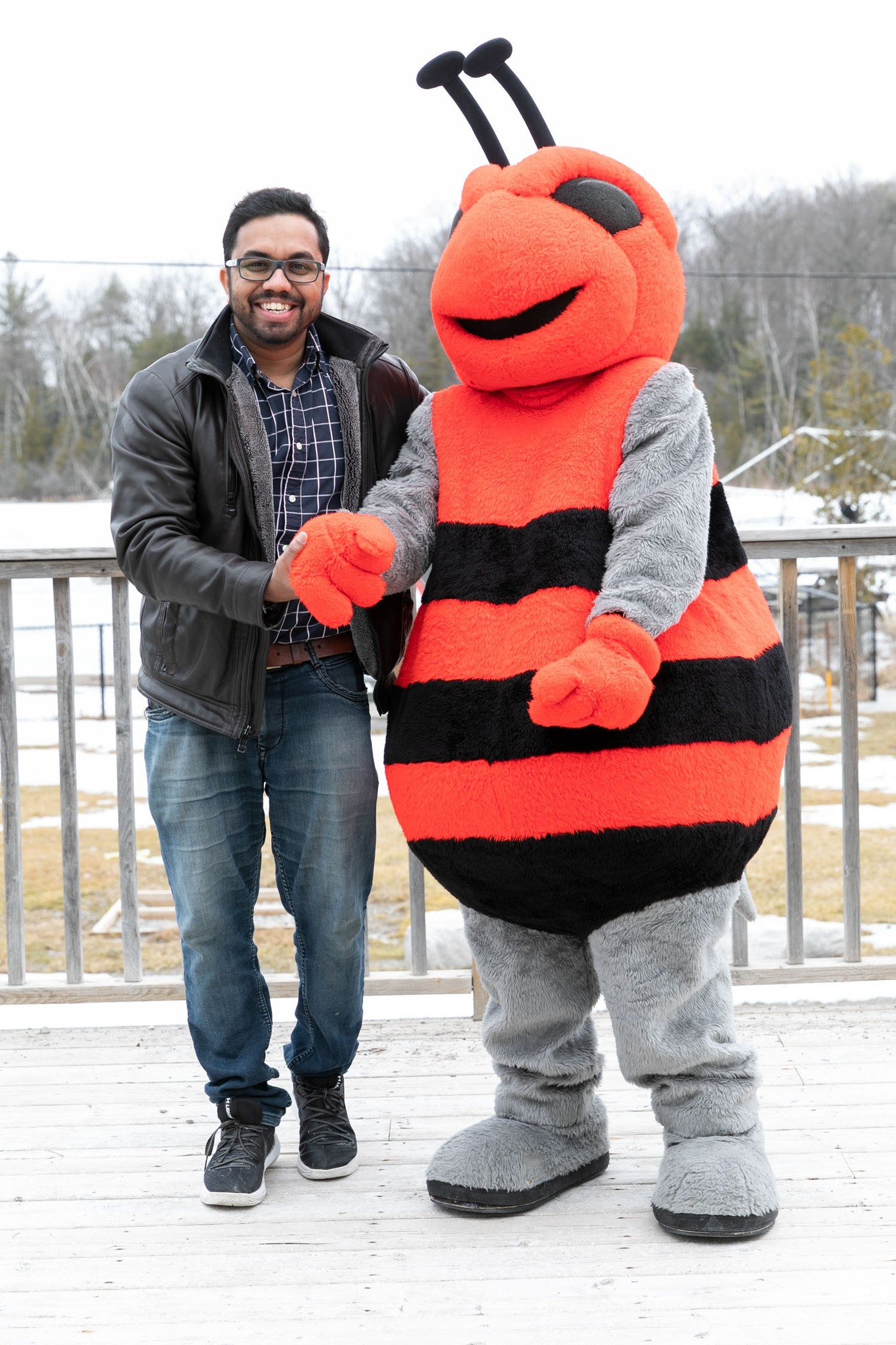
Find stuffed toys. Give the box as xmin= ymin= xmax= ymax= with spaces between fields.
xmin=288 ymin=37 xmax=794 ymax=1237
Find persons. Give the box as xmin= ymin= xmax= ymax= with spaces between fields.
xmin=110 ymin=186 xmax=432 ymax=1206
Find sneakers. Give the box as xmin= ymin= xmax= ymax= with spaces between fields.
xmin=199 ymin=1118 xmax=281 ymax=1208
xmin=290 ymin=1069 xmax=359 ymax=1180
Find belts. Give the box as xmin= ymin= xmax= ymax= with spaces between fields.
xmin=267 ymin=633 xmax=356 ymax=669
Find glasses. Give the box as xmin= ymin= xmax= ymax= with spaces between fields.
xmin=226 ymin=256 xmax=325 ymax=283
